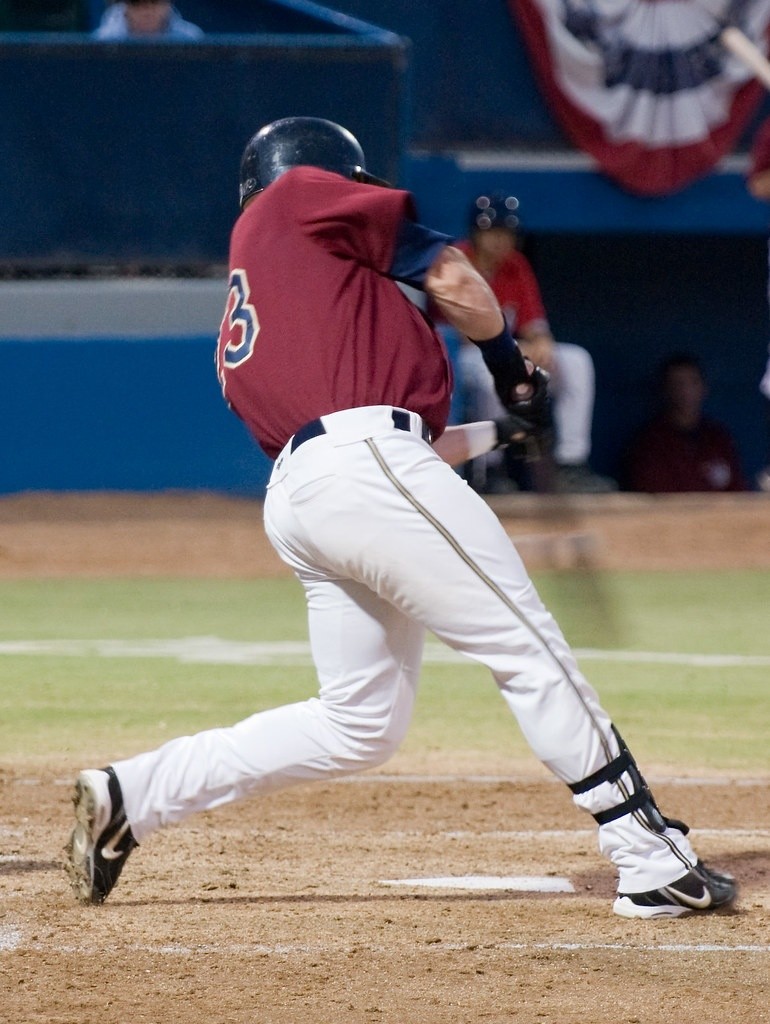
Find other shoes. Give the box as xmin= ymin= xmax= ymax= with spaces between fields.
xmin=561 ymin=464 xmax=617 ymax=493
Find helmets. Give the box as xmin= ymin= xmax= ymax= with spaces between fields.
xmin=470 ymin=196 xmax=517 ymax=230
xmin=239 ymin=116 xmax=394 ymax=213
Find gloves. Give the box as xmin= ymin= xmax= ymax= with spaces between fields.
xmin=490 ymin=410 xmax=555 ymax=451
xmin=481 ymin=342 xmax=551 ymax=416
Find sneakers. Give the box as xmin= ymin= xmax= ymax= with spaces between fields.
xmin=612 ymin=857 xmax=736 ymax=919
xmin=62 ymin=766 xmax=140 ymax=906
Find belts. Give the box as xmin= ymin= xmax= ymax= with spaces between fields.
xmin=290 ymin=410 xmax=433 ymax=454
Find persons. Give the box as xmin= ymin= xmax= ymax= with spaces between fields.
xmin=91 ymin=0 xmax=205 ymax=39
xmin=431 ymin=191 xmax=612 ymax=496
xmin=63 ymin=117 xmax=736 ymax=919
xmin=623 ymin=352 xmax=741 ymax=491
